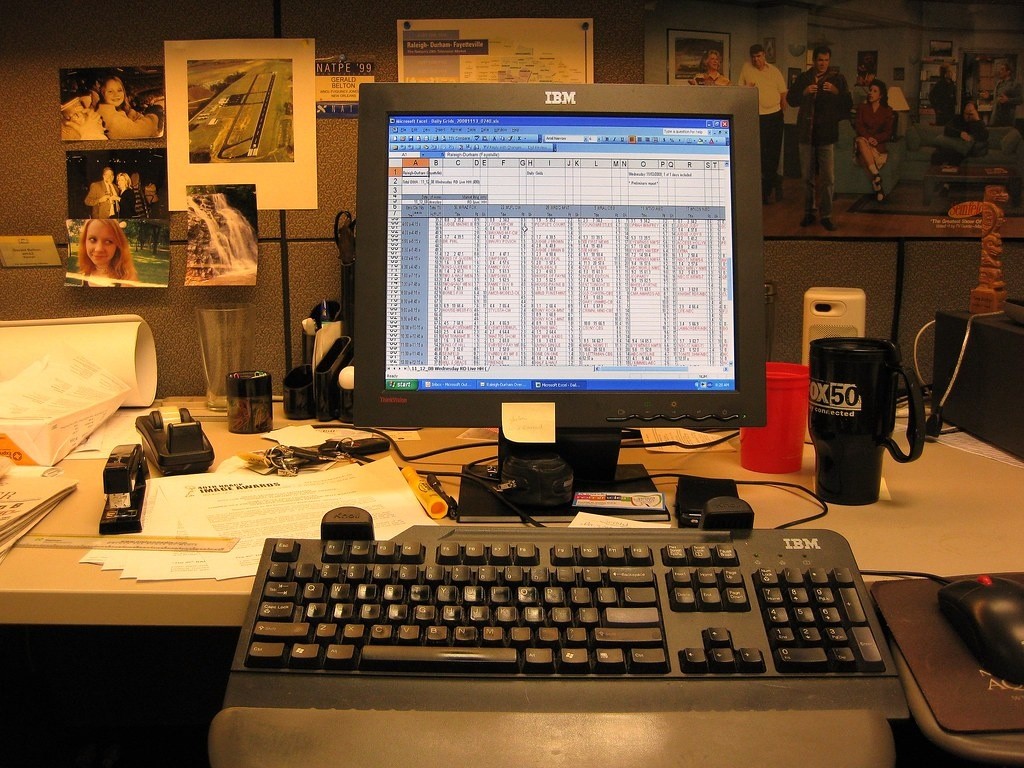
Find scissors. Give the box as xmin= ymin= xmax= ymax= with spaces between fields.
xmin=334 ymin=210 xmax=356 ymax=264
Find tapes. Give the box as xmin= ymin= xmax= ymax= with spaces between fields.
xmin=157 ymin=406 xmax=182 ymax=432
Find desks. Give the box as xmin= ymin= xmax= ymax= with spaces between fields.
xmin=923 ymin=167 xmax=1024 ymax=208
xmin=2 ymin=398 xmax=1024 ymax=630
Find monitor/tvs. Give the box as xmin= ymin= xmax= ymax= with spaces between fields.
xmin=351 ymin=84 xmax=766 ymax=518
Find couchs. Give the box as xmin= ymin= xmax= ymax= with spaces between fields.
xmin=810 ymin=120 xmax=1024 ymax=200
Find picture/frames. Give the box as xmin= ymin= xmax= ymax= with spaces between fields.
xmin=788 ymin=68 xmax=801 ymax=89
xmin=666 ymin=29 xmax=731 ymax=84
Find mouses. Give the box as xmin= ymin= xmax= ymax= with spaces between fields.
xmin=936 ymin=573 xmax=1024 ymax=688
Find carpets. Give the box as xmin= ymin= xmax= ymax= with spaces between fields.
xmin=846 ymin=180 xmax=1024 ymax=216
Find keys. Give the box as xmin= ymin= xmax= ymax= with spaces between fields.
xmin=261 ymin=436 xmax=388 ymax=477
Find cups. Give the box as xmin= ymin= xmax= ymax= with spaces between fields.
xmin=193 ymin=302 xmax=256 ymax=409
xmin=805 ymin=337 xmax=926 ymax=506
xmin=739 ymin=361 xmax=809 ymax=473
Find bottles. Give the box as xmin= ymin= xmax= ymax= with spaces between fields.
xmin=226 ymin=371 xmax=274 ymax=433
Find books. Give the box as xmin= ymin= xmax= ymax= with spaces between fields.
xmin=0 ymin=477 xmax=77 ymax=564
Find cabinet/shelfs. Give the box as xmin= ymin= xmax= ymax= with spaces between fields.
xmin=917 ymin=61 xmax=959 ymax=120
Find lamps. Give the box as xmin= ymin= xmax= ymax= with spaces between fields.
xmin=888 ymin=87 xmax=910 ymax=140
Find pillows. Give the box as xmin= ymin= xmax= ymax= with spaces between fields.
xmin=936 ymin=134 xmax=975 ymax=156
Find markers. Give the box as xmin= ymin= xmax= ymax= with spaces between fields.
xmin=401 ymin=464 xmax=449 ymax=520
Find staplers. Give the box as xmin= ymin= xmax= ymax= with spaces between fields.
xmin=98 ymin=443 xmax=150 ymax=536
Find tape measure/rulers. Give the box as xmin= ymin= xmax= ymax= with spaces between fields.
xmin=13 ymin=535 xmax=242 ymax=554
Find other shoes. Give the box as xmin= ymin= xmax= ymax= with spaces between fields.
xmin=872 ymin=173 xmax=882 ymax=186
xmin=821 ymin=217 xmax=838 ymax=231
xmin=875 ymin=187 xmax=886 ymax=204
xmin=801 ymin=214 xmax=816 ymax=227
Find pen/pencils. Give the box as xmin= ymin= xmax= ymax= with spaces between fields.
xmin=427 ymin=473 xmax=459 ymax=520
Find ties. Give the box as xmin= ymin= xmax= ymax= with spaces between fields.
xmin=109 ymin=185 xmax=113 ymax=197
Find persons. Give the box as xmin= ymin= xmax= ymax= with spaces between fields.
xmin=77 ymin=220 xmax=140 ymax=288
xmin=687 ymin=49 xmax=731 ymax=86
xmin=853 ymin=80 xmax=894 ymax=201
xmin=739 ymin=46 xmax=788 ymax=204
xmin=928 ymin=60 xmax=1024 ymax=193
xmin=61 ymin=77 xmax=159 ymax=141
xmin=786 ymin=48 xmax=852 ymax=232
xmin=85 ymin=168 xmax=158 ymax=219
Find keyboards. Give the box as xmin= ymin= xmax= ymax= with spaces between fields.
xmin=219 ymin=507 xmax=909 ymax=727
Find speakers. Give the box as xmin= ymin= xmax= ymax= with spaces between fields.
xmin=802 ymin=286 xmax=866 ymax=444
